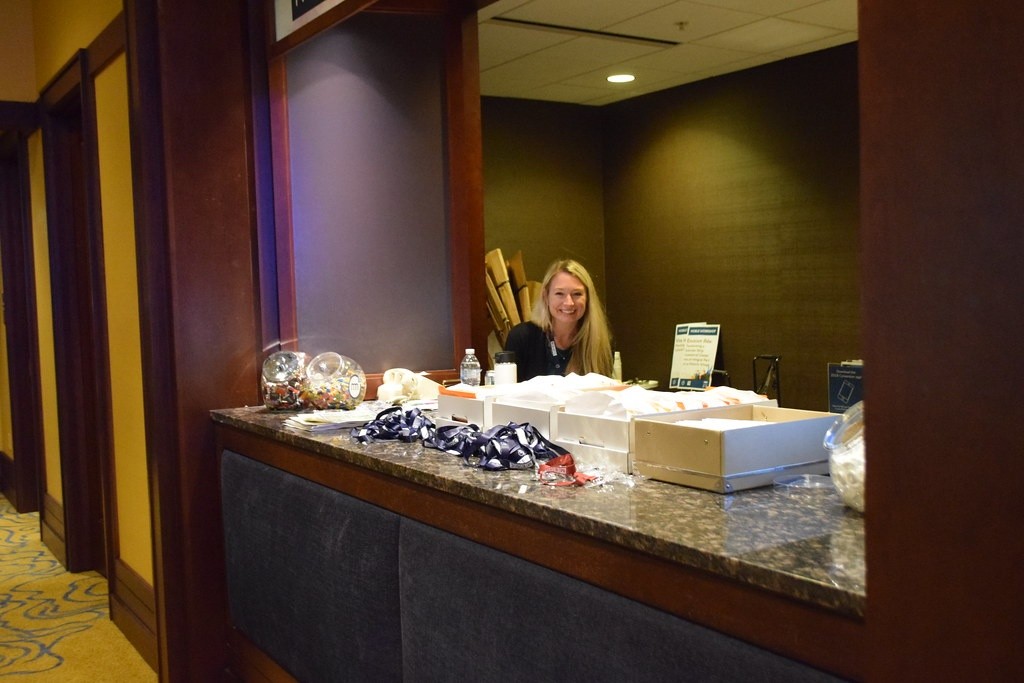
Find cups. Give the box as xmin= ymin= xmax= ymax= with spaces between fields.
xmin=494 ymin=352 xmax=518 ymax=386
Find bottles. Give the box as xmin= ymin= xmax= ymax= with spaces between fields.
xmin=484 ymin=370 xmax=495 ymax=385
xmin=459 ymin=348 xmax=481 ymax=386
xmin=612 ymin=352 xmax=622 ymax=381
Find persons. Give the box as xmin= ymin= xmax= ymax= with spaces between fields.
xmin=504 ymin=258 xmax=613 ymax=382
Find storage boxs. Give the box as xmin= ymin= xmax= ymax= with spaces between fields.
xmin=554 ymin=398 xmax=777 ymax=475
xmin=435 ymin=384 xmax=496 ymax=434
xmin=632 ymin=404 xmax=843 ymax=494
xmin=491 ymin=396 xmax=564 ymax=441
